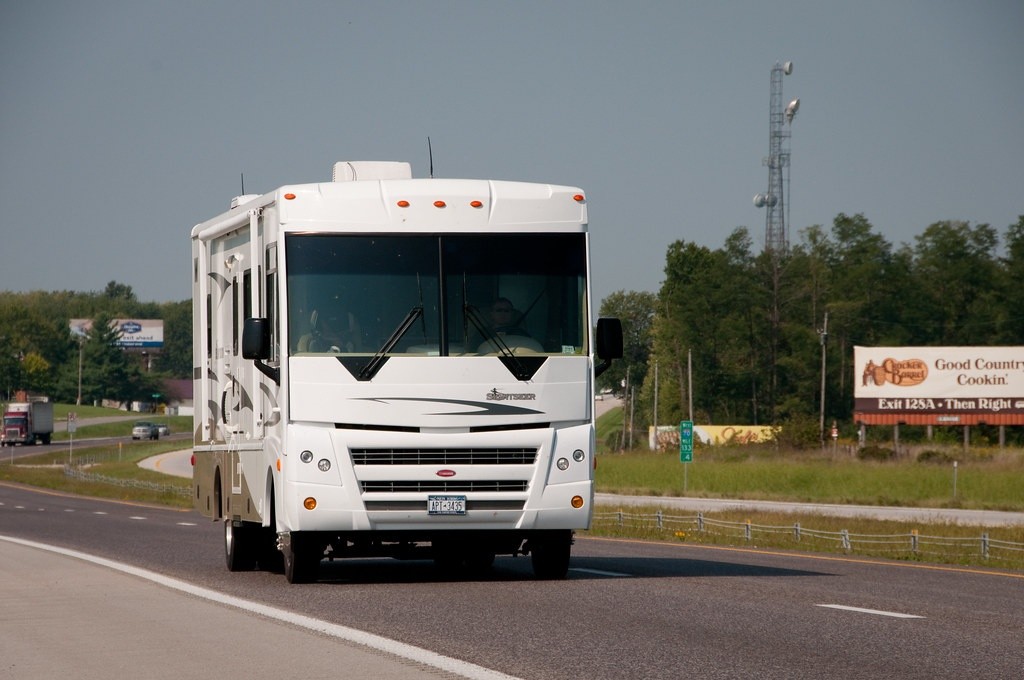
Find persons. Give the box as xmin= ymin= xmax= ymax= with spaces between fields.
xmin=470 ymin=298 xmax=532 ymax=350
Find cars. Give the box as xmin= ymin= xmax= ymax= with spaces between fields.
xmin=131 ymin=421 xmax=160 ymax=440
xmin=155 ymin=423 xmax=170 ymax=437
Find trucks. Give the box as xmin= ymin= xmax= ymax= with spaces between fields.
xmin=0 ymin=395 xmax=54 ymax=447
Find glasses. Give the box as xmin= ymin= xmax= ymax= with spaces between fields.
xmin=494 ymin=308 xmax=511 ymax=312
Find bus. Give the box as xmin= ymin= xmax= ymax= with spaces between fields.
xmin=191 ymin=161 xmax=623 ymax=585
